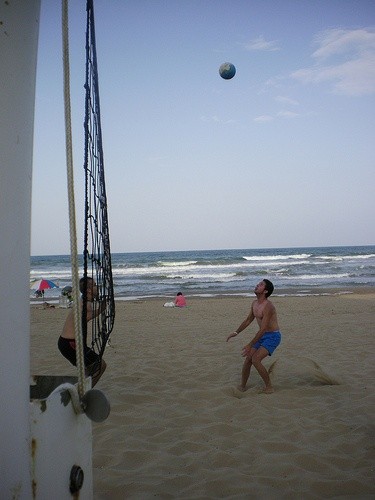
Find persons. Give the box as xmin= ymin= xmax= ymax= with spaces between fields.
xmin=174 ymin=292 xmax=186 ymax=307
xmin=226 ymin=279 xmax=281 ymax=393
xmin=58 ymin=277 xmax=107 ymax=389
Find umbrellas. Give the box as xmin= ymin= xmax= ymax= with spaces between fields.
xmin=30 ymin=278 xmax=59 ymax=302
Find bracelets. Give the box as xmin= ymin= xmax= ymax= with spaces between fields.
xmin=234 ymin=331 xmax=239 ymax=335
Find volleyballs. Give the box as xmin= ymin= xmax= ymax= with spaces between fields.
xmin=219 ymin=62 xmax=236 ymax=80
